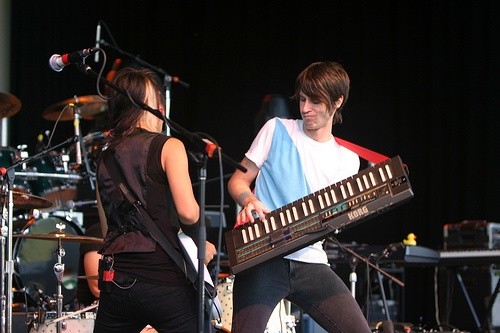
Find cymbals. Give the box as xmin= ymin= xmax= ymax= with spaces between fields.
xmin=0 ymin=188 xmax=53 ymax=209
xmin=0 ymin=88 xmax=22 ymax=120
xmin=10 ymin=231 xmax=105 ymax=244
xmin=73 ymin=273 xmax=100 ymax=282
xmin=38 ymin=94 xmax=110 ymax=123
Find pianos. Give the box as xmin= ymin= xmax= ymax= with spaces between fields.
xmin=224 ymin=155 xmax=414 ymax=276
xmin=437 ymin=248 xmax=500 ymax=333
xmin=324 ymin=242 xmax=440 ymax=333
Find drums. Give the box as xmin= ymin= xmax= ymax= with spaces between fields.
xmin=25 ymin=308 xmax=97 ymax=333
xmin=213 ymin=274 xmax=294 ymax=333
xmin=0 ymin=129 xmax=110 ymax=212
xmin=5 ymin=214 xmax=87 ymax=309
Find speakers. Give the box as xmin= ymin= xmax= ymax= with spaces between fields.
xmin=441 ymin=222 xmax=500 ymax=333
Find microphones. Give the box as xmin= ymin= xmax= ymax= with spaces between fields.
xmin=95 ymin=25 xmax=101 ymax=63
xmin=66 ymin=199 xmax=97 ymax=210
xmin=74 ymin=108 xmax=81 ymax=165
xmin=49 ymin=47 xmax=99 ymax=71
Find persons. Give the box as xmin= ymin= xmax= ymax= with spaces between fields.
xmin=78 ymin=221 xmax=109 ymax=309
xmin=228 ymin=61 xmax=372 ymax=333
xmin=92 ymin=66 xmax=217 ymax=333
